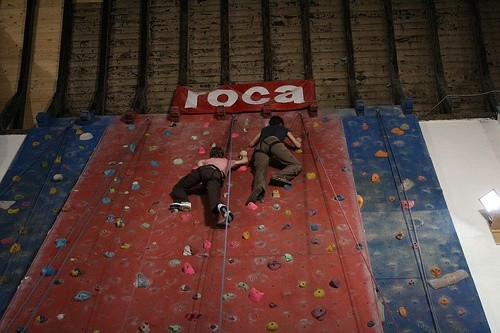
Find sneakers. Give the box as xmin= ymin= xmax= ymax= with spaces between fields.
xmin=247 ymin=187 xmax=265 ymax=204
xmin=218 ymin=204 xmax=234 ymax=223
xmin=170 ymin=202 xmax=192 ymax=211
xmin=270 ymin=176 xmax=293 ymax=186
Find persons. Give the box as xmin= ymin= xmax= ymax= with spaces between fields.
xmin=246 ymin=116 xmax=302 ymax=206
xmin=169 ymin=147 xmax=248 ymax=223
